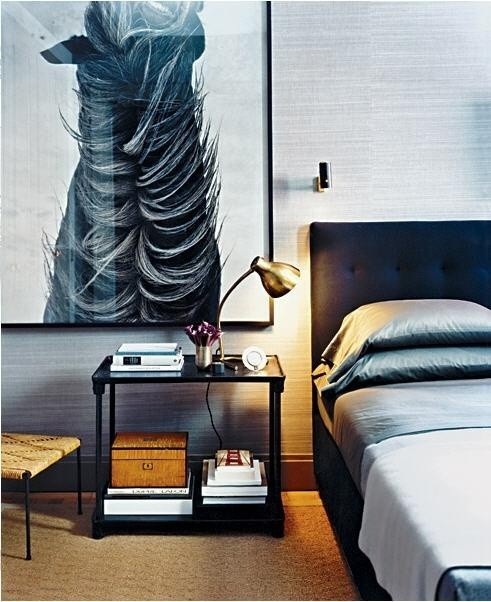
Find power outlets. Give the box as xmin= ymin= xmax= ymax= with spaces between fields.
xmin=317 ymin=176 xmax=328 ymax=192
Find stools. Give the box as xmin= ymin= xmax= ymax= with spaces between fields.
xmin=2 ymin=432 xmax=82 ymax=560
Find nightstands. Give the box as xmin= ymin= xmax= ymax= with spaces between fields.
xmin=91 ymin=354 xmax=287 ymax=540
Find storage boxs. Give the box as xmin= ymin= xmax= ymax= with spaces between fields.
xmin=112 ymin=433 xmax=187 ymax=488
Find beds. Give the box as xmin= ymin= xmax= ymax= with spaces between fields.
xmin=309 ymin=220 xmax=490 ymax=601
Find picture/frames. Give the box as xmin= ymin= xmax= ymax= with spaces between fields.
xmin=1 ymin=1 xmax=274 ymax=330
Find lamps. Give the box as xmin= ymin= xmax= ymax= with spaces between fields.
xmin=215 ymin=255 xmax=300 ymax=372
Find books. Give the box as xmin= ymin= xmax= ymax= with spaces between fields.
xmin=200 ymin=449 xmax=269 ymax=508
xmin=109 ymin=469 xmax=193 ymax=494
xmin=110 ymin=342 xmax=185 ymax=372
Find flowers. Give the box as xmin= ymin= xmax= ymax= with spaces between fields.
xmin=185 ymin=322 xmax=223 ymax=346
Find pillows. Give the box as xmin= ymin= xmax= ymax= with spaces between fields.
xmin=320 ymin=300 xmax=491 ymax=383
xmin=312 ymin=346 xmax=491 ymax=400
